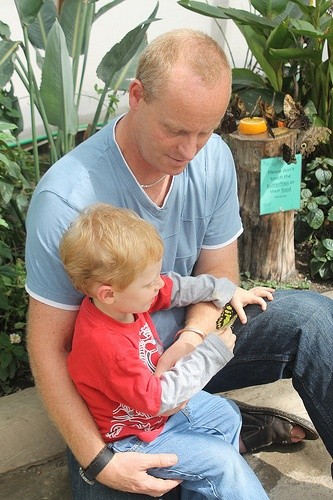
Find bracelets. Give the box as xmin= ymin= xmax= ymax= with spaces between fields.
xmin=172 ymin=328 xmax=208 ymax=339
xmin=79 ymin=446 xmax=114 ymax=485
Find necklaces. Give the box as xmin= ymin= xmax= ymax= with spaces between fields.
xmin=140 ymin=174 xmax=167 ymax=188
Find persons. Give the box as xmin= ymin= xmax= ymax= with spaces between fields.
xmin=61 ymin=203 xmax=275 ymax=500
xmin=24 ymin=29 xmax=333 ymax=500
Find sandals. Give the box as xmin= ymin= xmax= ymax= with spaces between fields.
xmin=230 ymin=398 xmax=320 ymax=454
